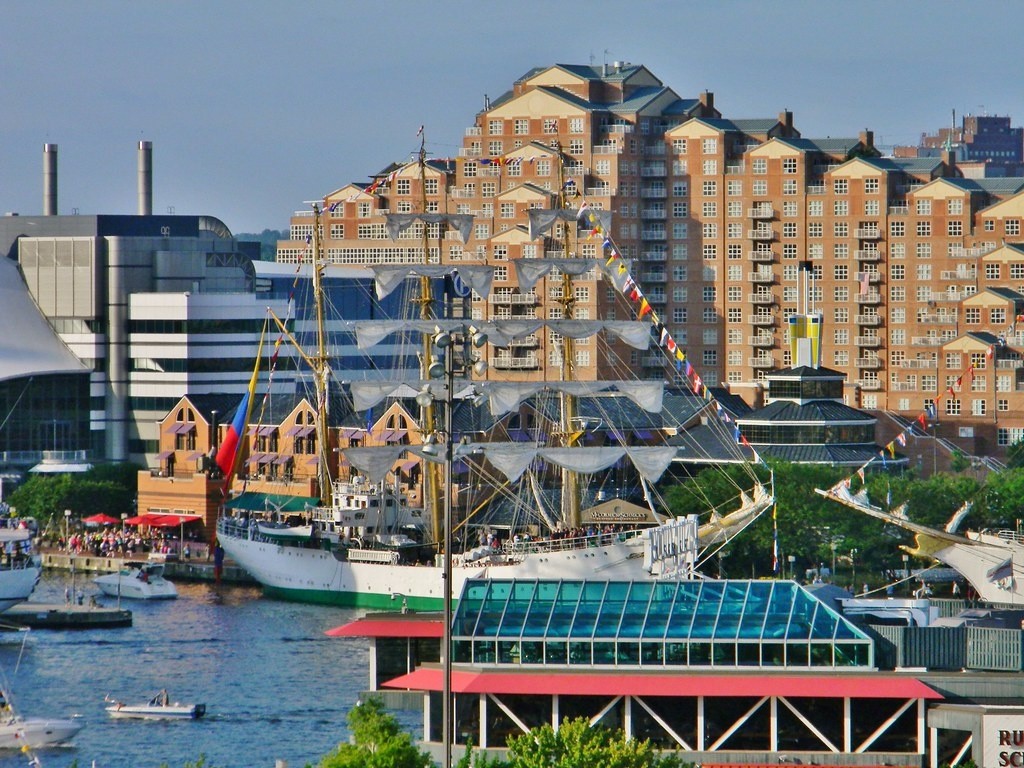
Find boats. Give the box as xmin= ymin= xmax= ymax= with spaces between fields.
xmin=0 ymin=713 xmax=89 ymax=749
xmin=104 ymin=691 xmax=207 ymax=720
xmin=0 ymin=528 xmax=42 ymax=616
xmin=92 ymin=561 xmax=178 ymax=601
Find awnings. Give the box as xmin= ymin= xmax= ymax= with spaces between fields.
xmin=154 ymin=423 xmax=205 ymax=461
xmin=339 ymin=429 xmax=418 ymax=471
xmin=381 ymin=668 xmax=944 ymax=700
xmin=124 ymin=513 xmax=201 ymax=527
xmin=452 ymin=429 xmax=471 ymax=473
xmin=245 ymin=426 xmax=319 ymax=465
xmin=224 ymin=491 xmax=321 ymax=510
xmin=627 ymin=430 xmax=652 ymax=441
xmin=508 ymin=428 xmax=549 ymax=471
xmin=582 ymin=430 xmax=620 ymax=441
xmin=322 ymin=621 xmax=444 ymax=637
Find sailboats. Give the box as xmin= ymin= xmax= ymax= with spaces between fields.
xmin=214 ymin=126 xmax=774 ymax=617
xmin=811 ymin=486 xmax=1024 ymax=605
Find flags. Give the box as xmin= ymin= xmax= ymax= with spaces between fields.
xmin=252 ymin=233 xmax=313 ymax=451
xmin=564 ymin=178 xmax=769 ymax=471
xmin=830 ymin=321 xmax=1015 ymax=496
xmin=318 ymin=156 xmax=536 ymax=213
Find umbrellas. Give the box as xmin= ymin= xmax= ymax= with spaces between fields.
xmin=83 ymin=514 xmax=120 ymax=523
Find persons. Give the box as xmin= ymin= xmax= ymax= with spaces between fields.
xmin=65 ymin=585 xmax=70 ymax=604
xmin=479 ymin=525 xmax=636 ymax=552
xmin=478 ymin=561 xmax=482 ymax=567
xmin=223 ymin=513 xmax=316 ymax=546
xmin=57 ymin=524 xmax=210 ymax=563
xmin=414 ymin=557 xmax=432 ymax=567
xmin=0 ymin=502 xmax=34 ymax=565
xmin=805 ymin=563 xmax=975 ymax=600
xmin=161 ymin=688 xmax=169 ymax=707
xmin=76 ymin=588 xmax=97 ymax=611
xmin=353 ymin=473 xmax=376 ymax=495
